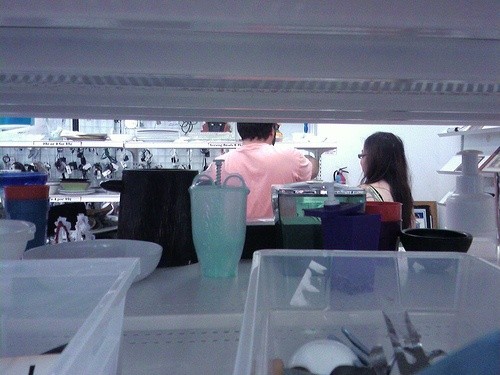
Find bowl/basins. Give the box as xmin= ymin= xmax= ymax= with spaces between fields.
xmin=22 ymin=239 xmax=163 ymax=285
xmin=44 ymin=182 xmax=59 ymax=194
xmin=61 ymin=183 xmax=89 ymax=189
xmin=401 ymin=228 xmax=473 ymax=253
xmin=0 ymin=218 xmax=36 ymax=294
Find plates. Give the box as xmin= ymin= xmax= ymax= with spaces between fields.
xmin=1 ymin=124 xmax=234 ymax=141
xmin=58 ymin=178 xmax=122 ymax=196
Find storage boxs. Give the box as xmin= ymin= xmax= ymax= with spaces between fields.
xmin=270 ymin=185 xmax=368 ymax=275
xmin=232 ymin=249 xmax=500 ymax=375
xmin=0 ymin=258 xmax=139 ymax=375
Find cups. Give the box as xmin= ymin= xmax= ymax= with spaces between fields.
xmin=0 ymin=169 xmax=50 ymax=250
xmin=188 ymin=173 xmax=251 ymax=279
xmin=322 ymin=201 xmax=403 ymax=294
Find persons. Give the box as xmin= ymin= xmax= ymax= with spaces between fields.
xmin=338 ymin=129 xmax=417 ymax=247
xmin=198 ymin=120 xmax=317 ymax=219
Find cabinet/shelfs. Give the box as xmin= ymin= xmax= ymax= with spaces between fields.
xmin=437 ymin=126 xmax=500 ymax=208
xmin=0 ymin=139 xmax=337 ymax=206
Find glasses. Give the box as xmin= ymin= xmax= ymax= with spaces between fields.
xmin=274 ymin=123 xmax=281 ymax=130
xmin=358 ymin=153 xmax=367 ymax=159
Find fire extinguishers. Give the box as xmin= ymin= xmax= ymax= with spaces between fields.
xmin=336 ymin=167 xmax=349 ymax=184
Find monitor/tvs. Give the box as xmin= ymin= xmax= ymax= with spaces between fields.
xmin=398 ymin=199 xmax=439 ymax=248
